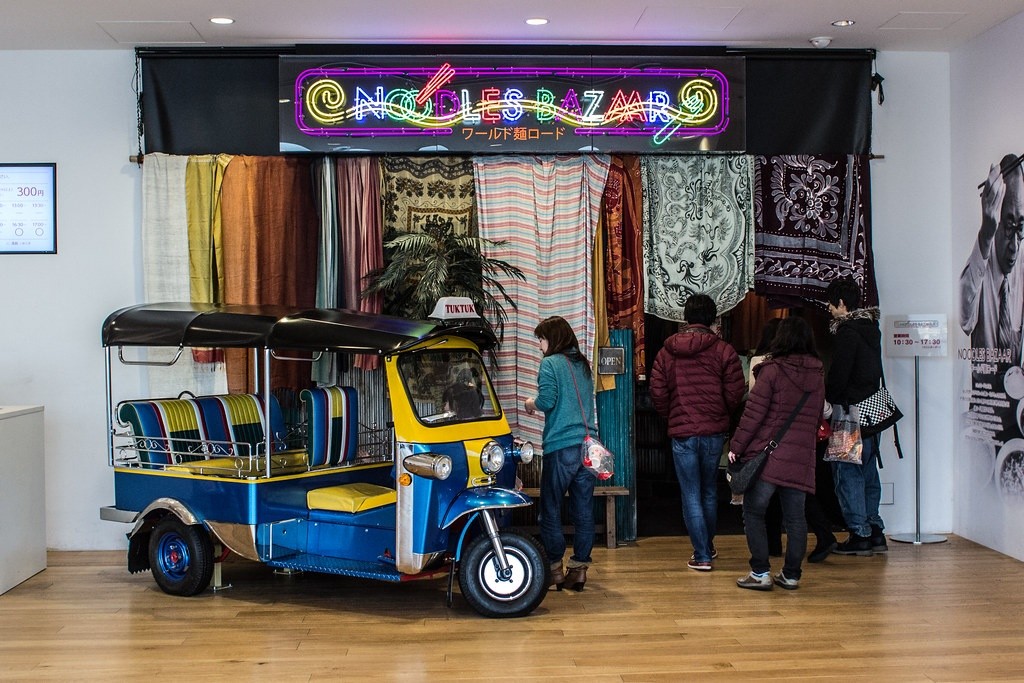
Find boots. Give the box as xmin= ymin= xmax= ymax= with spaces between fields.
xmin=549 ymin=559 xmax=565 ymax=591
xmin=765 ymin=488 xmax=783 ymax=556
xmin=805 ymin=493 xmax=838 ymax=562
xmin=563 ymin=556 xmax=592 ymax=591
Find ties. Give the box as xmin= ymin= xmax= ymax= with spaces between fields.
xmin=997 ymin=276 xmax=1012 ymax=374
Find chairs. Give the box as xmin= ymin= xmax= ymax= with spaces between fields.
xmin=300 ymin=387 xmax=397 ymax=513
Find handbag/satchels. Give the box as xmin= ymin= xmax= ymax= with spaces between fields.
xmin=822 ymin=405 xmax=863 ymax=465
xmin=581 ymin=436 xmax=614 ymax=480
xmin=853 ymin=387 xmax=904 ymax=438
xmin=726 ymin=440 xmax=778 ymax=494
xmin=815 ymin=413 xmax=832 ymax=442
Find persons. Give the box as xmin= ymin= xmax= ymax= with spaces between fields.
xmin=749 ymin=316 xmax=837 ymax=563
xmin=821 ymin=278 xmax=889 ymax=557
xmin=649 ymin=294 xmax=746 ymax=569
xmin=959 ymin=153 xmax=1024 ymax=439
xmin=727 ymin=317 xmax=825 ymax=591
xmin=525 ymin=316 xmax=598 ymax=592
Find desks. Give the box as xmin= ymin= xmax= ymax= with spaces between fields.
xmin=0 ymin=405 xmax=47 ymax=596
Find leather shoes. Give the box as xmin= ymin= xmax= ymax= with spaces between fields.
xmin=871 ymin=525 xmax=888 ymax=552
xmin=832 ymin=530 xmax=872 ymax=556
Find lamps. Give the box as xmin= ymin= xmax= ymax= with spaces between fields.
xmin=808 ymin=36 xmax=833 ymax=48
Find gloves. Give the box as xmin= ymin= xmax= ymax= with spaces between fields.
xmin=835 ymin=396 xmax=849 ymax=414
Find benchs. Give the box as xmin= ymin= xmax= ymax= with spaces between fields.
xmin=524 ymin=486 xmax=629 ymax=549
xmin=116 ymin=391 xmax=325 ymax=479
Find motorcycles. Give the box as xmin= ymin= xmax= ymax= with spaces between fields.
xmin=99 ymin=295 xmax=553 ymax=621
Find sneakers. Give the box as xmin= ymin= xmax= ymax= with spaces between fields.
xmin=773 ymin=569 xmax=798 ymax=589
xmin=691 ymin=549 xmax=717 ymax=559
xmin=688 ymin=560 xmax=712 ymax=569
xmin=737 ymin=571 xmax=772 ymax=589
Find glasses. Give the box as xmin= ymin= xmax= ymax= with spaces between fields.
xmin=1003 ymin=219 xmax=1024 ymax=240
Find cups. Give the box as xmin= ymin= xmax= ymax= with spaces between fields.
xmin=588 ymin=445 xmax=604 ymax=468
